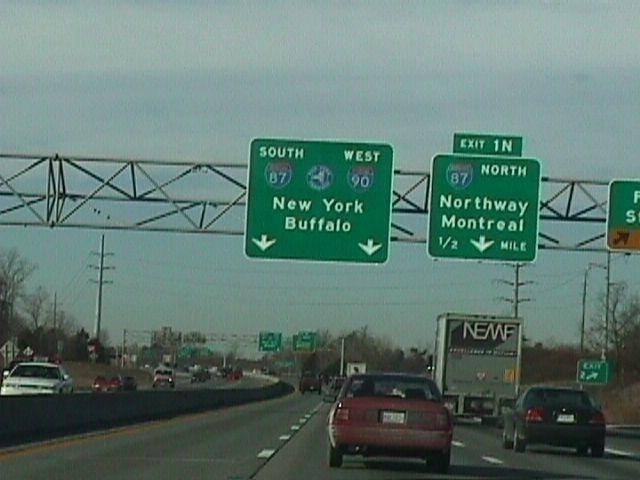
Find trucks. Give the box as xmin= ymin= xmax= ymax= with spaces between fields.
xmin=426 ymin=312 xmax=523 ymax=428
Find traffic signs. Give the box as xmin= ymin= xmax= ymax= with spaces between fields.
xmin=602 ymin=177 xmax=639 ymax=252
xmin=575 ymin=358 xmax=609 ymax=388
xmin=424 ymin=133 xmax=543 ymax=264
xmin=242 ymin=136 xmax=397 ymax=264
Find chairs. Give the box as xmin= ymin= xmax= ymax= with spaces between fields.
xmin=354 ymin=387 xmax=426 ymax=400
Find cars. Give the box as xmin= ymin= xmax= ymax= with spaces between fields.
xmin=92 ymin=373 xmax=137 ymax=392
xmin=1 ymin=360 xmax=74 ymax=395
xmin=497 ymin=383 xmax=608 ymax=458
xmin=325 ymin=370 xmax=453 ymax=473
